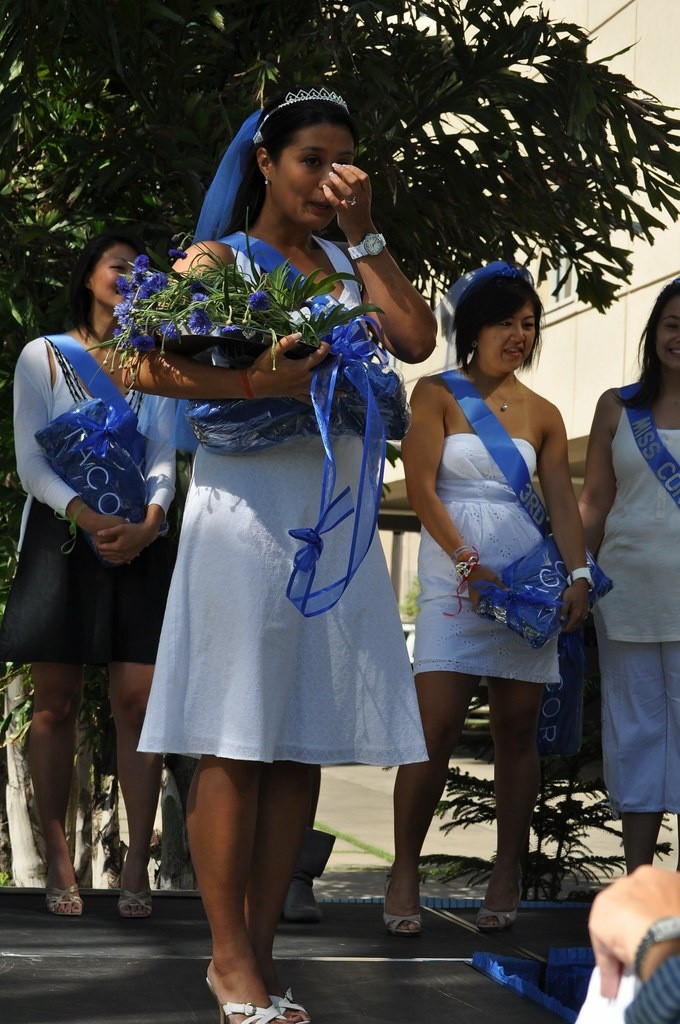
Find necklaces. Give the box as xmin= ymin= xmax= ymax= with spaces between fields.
xmin=467 ymin=364 xmax=517 ymax=411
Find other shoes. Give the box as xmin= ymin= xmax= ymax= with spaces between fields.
xmin=283 ymin=879 xmax=323 ymax=924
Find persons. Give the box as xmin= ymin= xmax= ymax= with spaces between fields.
xmin=575 ymin=863 xmax=680 ymax=1023
xmin=0 ymin=230 xmax=178 ymax=920
xmin=121 ymin=87 xmax=438 ymax=1024
xmin=579 ymin=276 xmax=680 ymax=875
xmin=383 ymin=262 xmax=595 ymax=936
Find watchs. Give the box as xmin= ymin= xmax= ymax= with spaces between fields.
xmin=347 ymin=233 xmax=387 ymax=260
xmin=634 ymin=916 xmax=680 ymax=979
xmin=567 ymin=567 xmax=595 ymax=592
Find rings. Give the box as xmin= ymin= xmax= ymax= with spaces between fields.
xmin=346 ymin=196 xmax=357 ymax=206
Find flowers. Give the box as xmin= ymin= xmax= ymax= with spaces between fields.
xmin=85 ymin=205 xmax=386 ymax=402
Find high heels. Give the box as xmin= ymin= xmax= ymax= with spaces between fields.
xmin=44 ymin=882 xmax=83 ymax=914
xmin=266 ymin=985 xmax=309 ymax=1024
xmin=474 ymin=886 xmax=523 ymax=930
xmin=383 ymin=866 xmax=423 ymax=938
xmin=116 ymin=889 xmax=153 ymax=917
xmin=206 ymin=974 xmax=287 ymax=1024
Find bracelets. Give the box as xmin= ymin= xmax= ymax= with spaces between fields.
xmin=240 ymin=368 xmax=256 ymax=400
xmin=54 ymin=503 xmax=88 ymax=555
xmin=443 ymin=546 xmax=480 ymax=618
